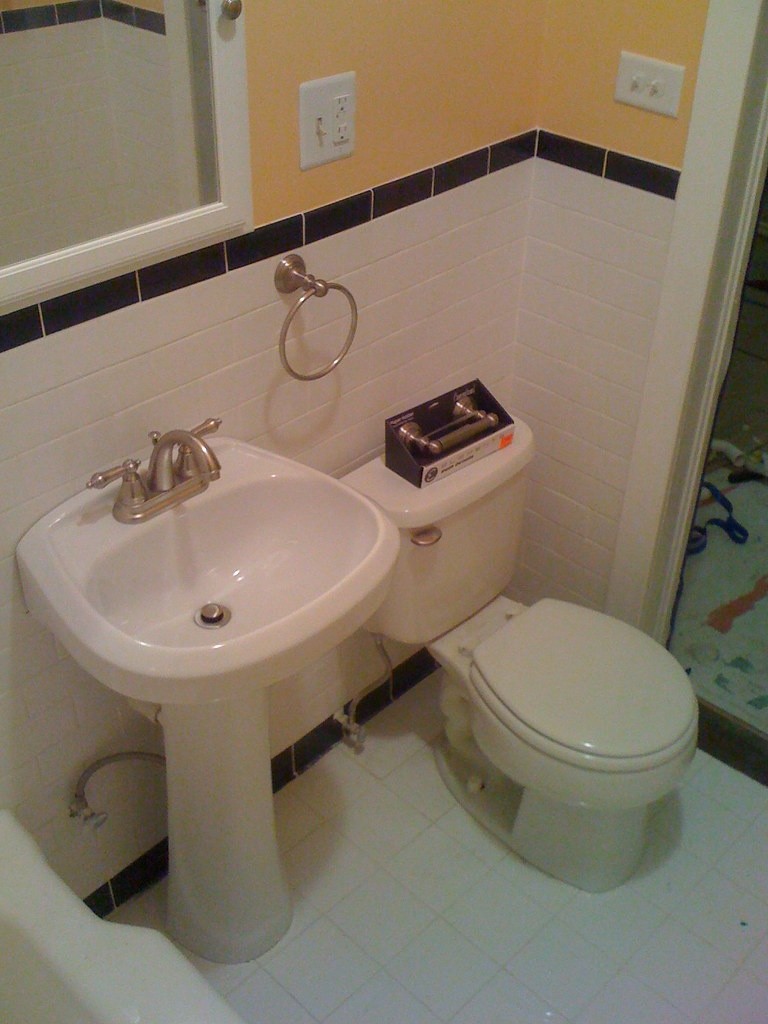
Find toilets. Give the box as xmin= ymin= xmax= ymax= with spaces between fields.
xmin=338 ymin=406 xmax=701 ymax=895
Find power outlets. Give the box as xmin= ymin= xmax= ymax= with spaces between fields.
xmin=300 ymin=72 xmax=357 ymax=171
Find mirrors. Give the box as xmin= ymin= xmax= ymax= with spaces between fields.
xmin=0 ymin=0 xmax=215 ymax=276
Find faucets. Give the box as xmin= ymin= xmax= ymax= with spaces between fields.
xmin=145 ymin=427 xmax=224 ymax=493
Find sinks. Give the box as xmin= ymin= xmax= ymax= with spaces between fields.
xmin=17 ymin=427 xmax=403 ymax=708
